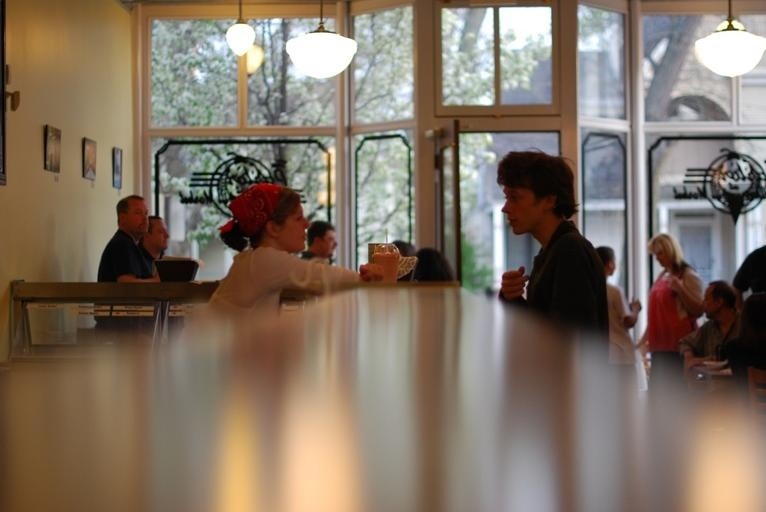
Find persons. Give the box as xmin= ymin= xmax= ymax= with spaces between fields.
xmin=410 ymin=248 xmax=452 ymax=281
xmin=391 ymin=238 xmax=420 ymax=282
xmin=679 ymin=280 xmax=744 ymax=377
xmin=731 ymin=241 xmax=766 ymax=294
xmin=633 ymin=232 xmax=706 ymax=413
xmin=301 ymin=219 xmax=337 ymax=266
xmin=138 ymin=213 xmax=169 ymax=260
xmin=95 ymin=194 xmax=168 ymax=337
xmin=493 ymin=149 xmax=608 ymax=351
xmin=197 ymin=183 xmax=354 ymax=337
xmin=595 ymin=244 xmax=642 ymax=405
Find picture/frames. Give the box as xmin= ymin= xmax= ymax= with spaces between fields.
xmin=80 ymin=136 xmax=97 ymax=182
xmin=111 ymin=147 xmax=123 ymax=190
xmin=43 ymin=124 xmax=62 ymax=173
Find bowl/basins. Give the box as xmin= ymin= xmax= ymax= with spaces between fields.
xmin=156 ymin=260 xmax=199 ymax=282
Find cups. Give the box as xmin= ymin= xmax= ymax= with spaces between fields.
xmin=373 ymin=245 xmax=402 ymax=284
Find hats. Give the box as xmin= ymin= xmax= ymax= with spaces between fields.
xmin=374 ymin=244 xmax=418 ymax=279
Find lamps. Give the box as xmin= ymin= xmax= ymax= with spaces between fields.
xmin=694 ymin=1 xmax=766 ymax=78
xmin=224 ymin=0 xmax=256 ymax=57
xmin=284 ymin=0 xmax=358 ymax=80
